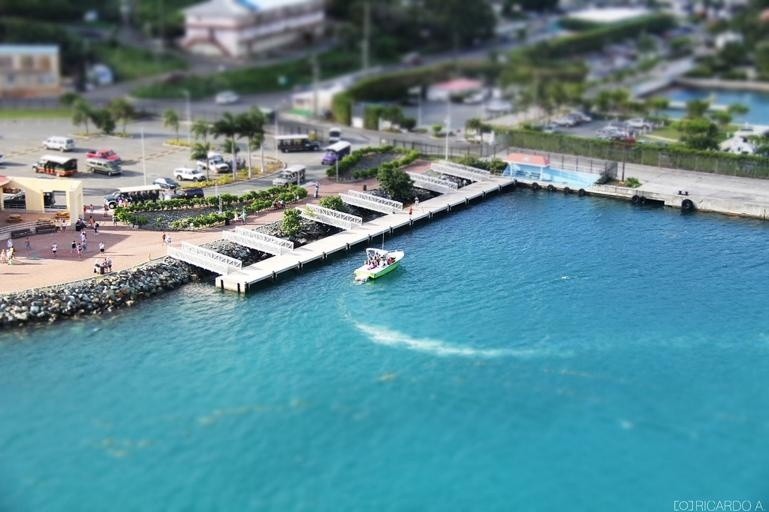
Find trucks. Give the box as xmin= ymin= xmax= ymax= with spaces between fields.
xmin=272 ymin=165 xmax=305 ymax=188
xmin=322 ymin=142 xmax=351 ymax=166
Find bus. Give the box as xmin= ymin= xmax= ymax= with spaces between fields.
xmin=102 ymin=187 xmax=164 ymax=208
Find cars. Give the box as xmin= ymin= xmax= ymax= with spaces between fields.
xmin=598 ymin=118 xmax=665 ymax=137
xmin=154 ymin=177 xmax=181 ymax=188
xmin=55 ymin=210 xmax=69 ymax=221
xmin=87 ymin=148 xmax=119 ymax=165
xmin=544 ymin=110 xmax=592 ymax=134
xmin=36 ymin=218 xmax=54 ymax=226
xmin=6 ymin=213 xmax=22 ymax=223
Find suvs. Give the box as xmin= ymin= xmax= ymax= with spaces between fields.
xmin=278 ymin=136 xmax=320 ymax=152
xmin=171 ymin=185 xmax=204 ymax=199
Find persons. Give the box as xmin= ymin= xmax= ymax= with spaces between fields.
xmin=0 ymin=238 xmax=16 ymax=266
xmin=25 ymin=237 xmax=32 ymax=250
xmin=278 ymin=198 xmax=285 ymax=207
xmin=162 ymin=190 xmax=167 ymax=200
xmin=71 ymin=195 xmax=133 ymax=273
xmin=276 ymin=200 xmax=283 ymax=209
xmin=162 ymin=233 xmax=165 ymax=244
xmin=368 ymin=253 xmax=387 ymax=266
xmin=50 ymin=242 xmax=58 ymax=256
xmin=235 ymin=212 xmax=238 ymax=222
xmin=413 ymin=196 xmax=420 ymax=206
xmin=408 ymin=206 xmax=415 ymax=220
xmin=293 ymin=191 xmax=298 ymax=204
xmin=240 ymin=209 xmax=248 ymax=225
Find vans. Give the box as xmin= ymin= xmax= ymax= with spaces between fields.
xmin=198 ymin=152 xmax=229 ymax=174
xmin=175 ymin=168 xmax=207 ymax=183
xmin=85 ymin=157 xmax=123 ymax=175
xmin=329 ymin=128 xmax=342 ymax=143
xmin=42 ymin=136 xmax=75 ymax=153
xmin=33 ymin=155 xmax=78 ymax=178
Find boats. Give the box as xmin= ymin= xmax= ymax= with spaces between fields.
xmin=354 ymin=248 xmax=405 ymax=279
